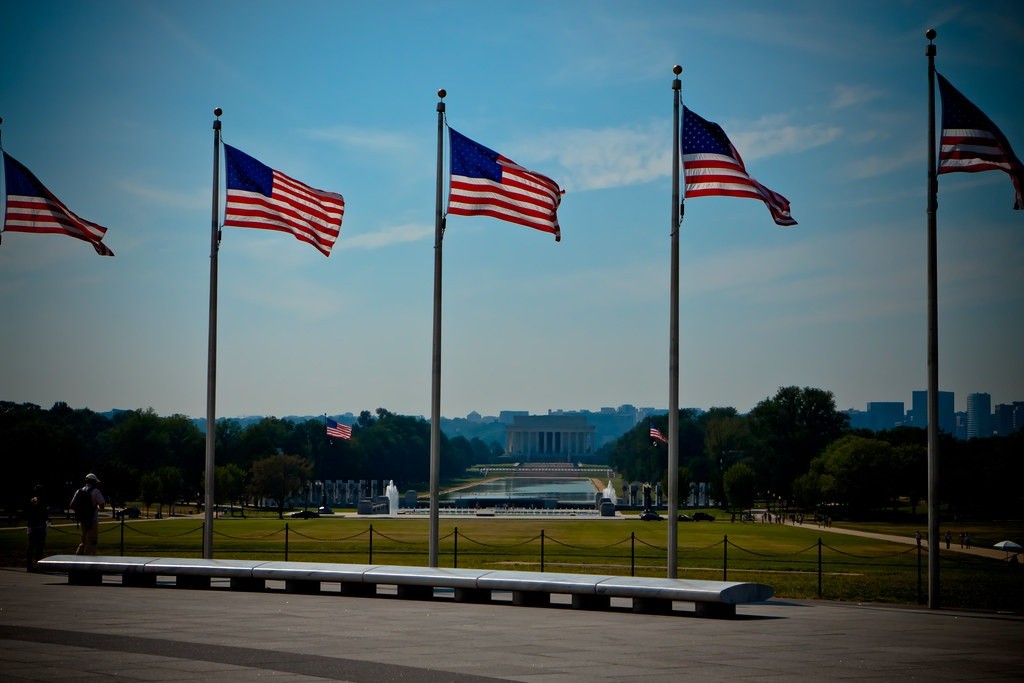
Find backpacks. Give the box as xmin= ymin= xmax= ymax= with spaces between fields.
xmin=69 ymin=487 xmax=96 ymax=525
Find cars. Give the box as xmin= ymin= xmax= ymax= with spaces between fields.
xmin=641 ymin=513 xmax=664 ymax=521
xmin=292 ymin=511 xmax=317 ymax=518
xmin=676 ymin=514 xmax=693 ymax=521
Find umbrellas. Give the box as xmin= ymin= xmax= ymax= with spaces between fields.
xmin=994 ymin=540 xmax=1022 ymax=561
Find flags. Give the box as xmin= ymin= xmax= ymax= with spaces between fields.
xmin=650 ymin=421 xmax=668 ymax=444
xmin=936 ymin=70 xmax=1024 ymax=210
xmin=2 ymin=149 xmax=114 ymax=256
xmin=682 ymin=104 xmax=799 ymax=226
xmin=447 ymin=125 xmax=565 ymax=242
xmin=327 ymin=418 xmax=352 ymax=441
xmin=223 ymin=142 xmax=345 ymax=257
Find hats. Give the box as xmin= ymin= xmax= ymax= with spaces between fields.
xmin=86 ymin=473 xmax=101 ymax=484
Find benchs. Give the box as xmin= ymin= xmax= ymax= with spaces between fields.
xmin=38 ymin=553 xmax=776 ymax=618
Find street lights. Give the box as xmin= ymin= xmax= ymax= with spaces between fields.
xmin=767 ymin=489 xmax=781 ymax=514
xmin=644 ymin=484 xmax=652 ymax=520
xmin=316 ymin=481 xmax=322 ymax=517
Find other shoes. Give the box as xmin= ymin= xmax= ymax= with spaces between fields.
xmin=26 ymin=566 xmax=37 ymax=573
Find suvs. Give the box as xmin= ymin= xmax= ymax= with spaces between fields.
xmin=116 ymin=507 xmax=141 ymax=517
xmin=692 ymin=512 xmax=715 ymax=521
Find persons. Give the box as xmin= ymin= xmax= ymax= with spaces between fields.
xmin=958 ymin=531 xmax=971 ymax=549
xmin=915 ymin=530 xmax=921 ymax=545
xmin=817 ymin=514 xmax=832 ymax=528
xmin=730 ymin=512 xmax=803 ymax=525
xmin=1010 ymin=555 xmax=1019 ymax=563
xmin=26 ymin=485 xmax=50 ymax=572
xmin=70 ymin=473 xmax=105 ymax=555
xmin=945 ymin=530 xmax=952 ymax=549
